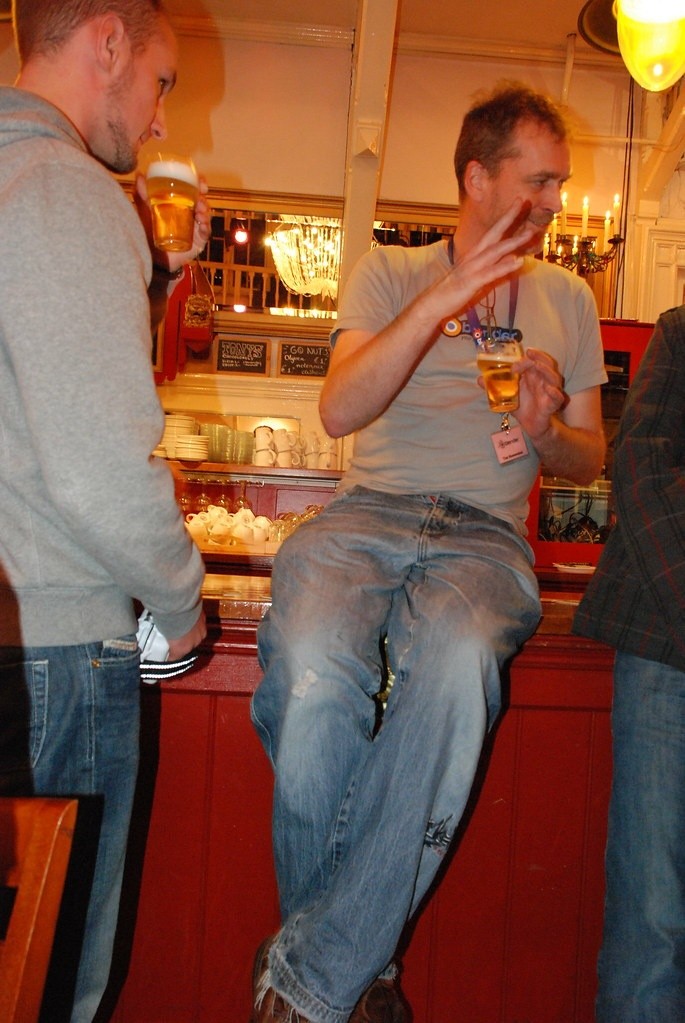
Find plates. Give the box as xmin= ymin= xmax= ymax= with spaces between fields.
xmin=152 ymin=415 xmax=253 ymax=465
xmin=555 ymin=564 xmax=596 ymax=574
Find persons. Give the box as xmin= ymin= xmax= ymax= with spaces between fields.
xmin=572 ymin=300 xmax=684 ymax=1022
xmin=245 ymin=89 xmax=607 ymax=1023
xmin=0 ymin=0 xmax=211 ymax=1023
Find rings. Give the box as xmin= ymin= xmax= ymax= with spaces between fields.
xmin=195 ymin=218 xmax=201 ymax=225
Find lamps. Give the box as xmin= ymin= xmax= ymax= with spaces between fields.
xmin=577 ymin=0 xmax=685 ymax=92
xmin=262 ymin=214 xmax=395 ymax=298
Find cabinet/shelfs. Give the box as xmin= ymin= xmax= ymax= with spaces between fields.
xmin=523 ymin=316 xmax=656 ymax=586
xmin=112 ymin=457 xmax=619 ymax=1023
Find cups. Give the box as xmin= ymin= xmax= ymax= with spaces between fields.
xmin=146 ymin=151 xmax=199 ymax=253
xmin=184 ymin=504 xmax=323 ymax=545
xmin=477 ymin=339 xmax=523 ymax=413
xmin=252 ymin=427 xmax=338 ymax=471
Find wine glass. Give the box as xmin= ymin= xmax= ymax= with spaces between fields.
xmin=178 ymin=480 xmax=253 ymax=511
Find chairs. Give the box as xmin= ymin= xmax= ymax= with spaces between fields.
xmin=0 ymin=795 xmax=78 ymax=1023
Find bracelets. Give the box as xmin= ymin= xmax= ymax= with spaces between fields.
xmin=152 ymin=264 xmax=183 ymax=280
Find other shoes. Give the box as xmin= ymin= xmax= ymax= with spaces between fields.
xmin=348 ymin=960 xmax=410 ymax=1023
xmin=251 ymin=935 xmax=312 ymax=1023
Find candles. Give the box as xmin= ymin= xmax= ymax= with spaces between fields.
xmin=614 ymin=193 xmax=621 ymax=234
xmin=560 ymin=192 xmax=568 ymax=235
xmin=551 ymin=213 xmax=557 ymax=252
xmin=571 ymin=235 xmax=579 ymax=255
xmin=581 ymin=196 xmax=589 ymax=237
xmin=542 ymin=232 xmax=550 ymax=263
xmin=603 ymin=210 xmax=610 ymax=252
